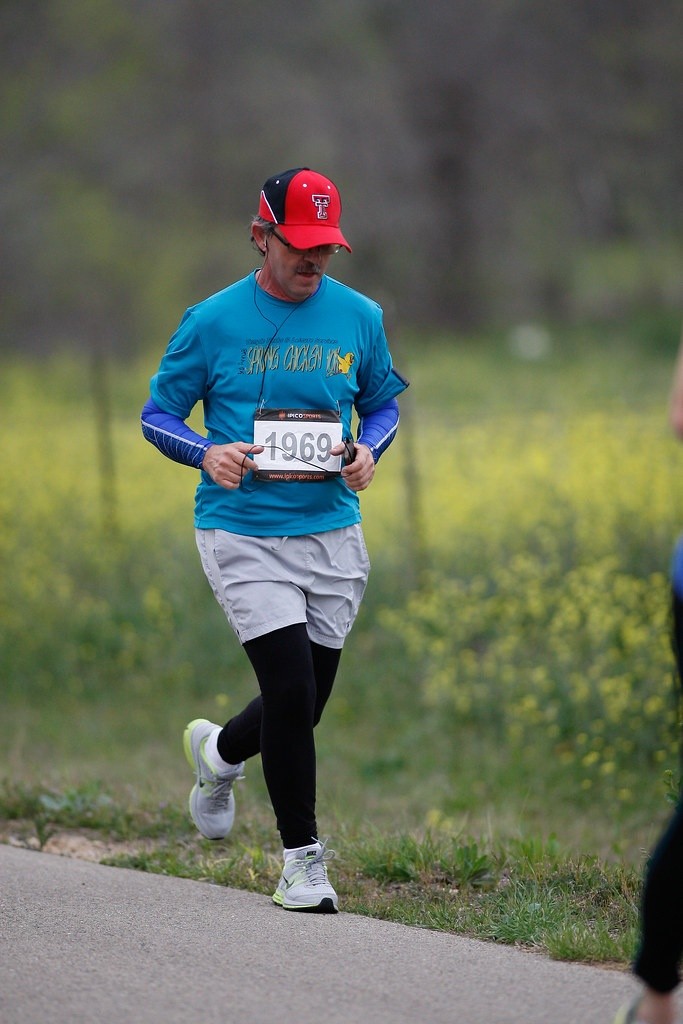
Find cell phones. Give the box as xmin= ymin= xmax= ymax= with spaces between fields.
xmin=343 ymin=440 xmax=356 ymax=466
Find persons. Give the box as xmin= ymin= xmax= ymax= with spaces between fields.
xmin=616 ymin=346 xmax=683 ymax=1024
xmin=141 ymin=166 xmax=413 ymax=914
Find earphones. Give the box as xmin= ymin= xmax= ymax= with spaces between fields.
xmin=263 ymin=233 xmax=268 ymax=247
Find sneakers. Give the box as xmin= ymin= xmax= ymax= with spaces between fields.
xmin=182 ymin=718 xmax=246 ymax=839
xmin=272 ymin=836 xmax=340 ymax=914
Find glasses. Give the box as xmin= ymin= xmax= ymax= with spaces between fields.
xmin=270 ymin=227 xmax=343 ymax=254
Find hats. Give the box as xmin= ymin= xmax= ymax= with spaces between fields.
xmin=258 ymin=167 xmax=353 ymax=254
